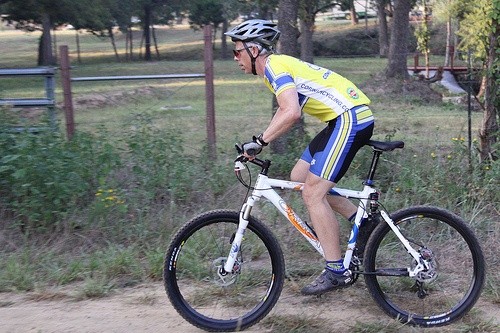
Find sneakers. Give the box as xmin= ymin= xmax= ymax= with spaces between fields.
xmin=353 ymin=214 xmax=380 ymax=256
xmin=301 ymin=267 xmax=353 ymax=296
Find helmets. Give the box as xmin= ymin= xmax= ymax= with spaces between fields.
xmin=224 ymin=18 xmax=281 ymax=46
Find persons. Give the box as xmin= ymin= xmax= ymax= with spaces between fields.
xmin=223 ymin=19 xmax=375 ymax=294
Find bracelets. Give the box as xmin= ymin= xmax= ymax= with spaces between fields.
xmin=256 ymin=133 xmax=269 ymax=146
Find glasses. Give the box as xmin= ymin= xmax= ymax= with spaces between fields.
xmin=232 ymin=46 xmax=253 ymax=56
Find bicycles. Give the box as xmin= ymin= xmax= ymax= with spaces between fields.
xmin=160 ymin=133 xmax=487 ymax=332
